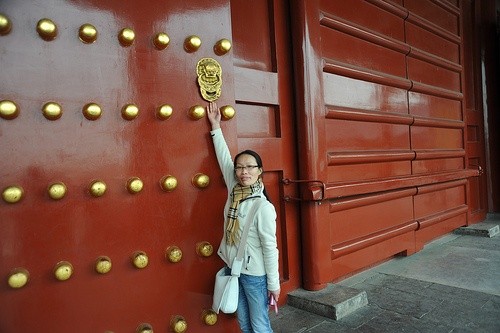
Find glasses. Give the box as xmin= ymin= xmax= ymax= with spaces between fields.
xmin=234 ymin=165 xmax=260 ymax=170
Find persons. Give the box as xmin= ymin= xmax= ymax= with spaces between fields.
xmin=206 ymin=100 xmax=280 ymax=333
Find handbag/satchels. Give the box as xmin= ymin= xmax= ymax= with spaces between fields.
xmin=211 ymin=266 xmax=239 ymax=314
